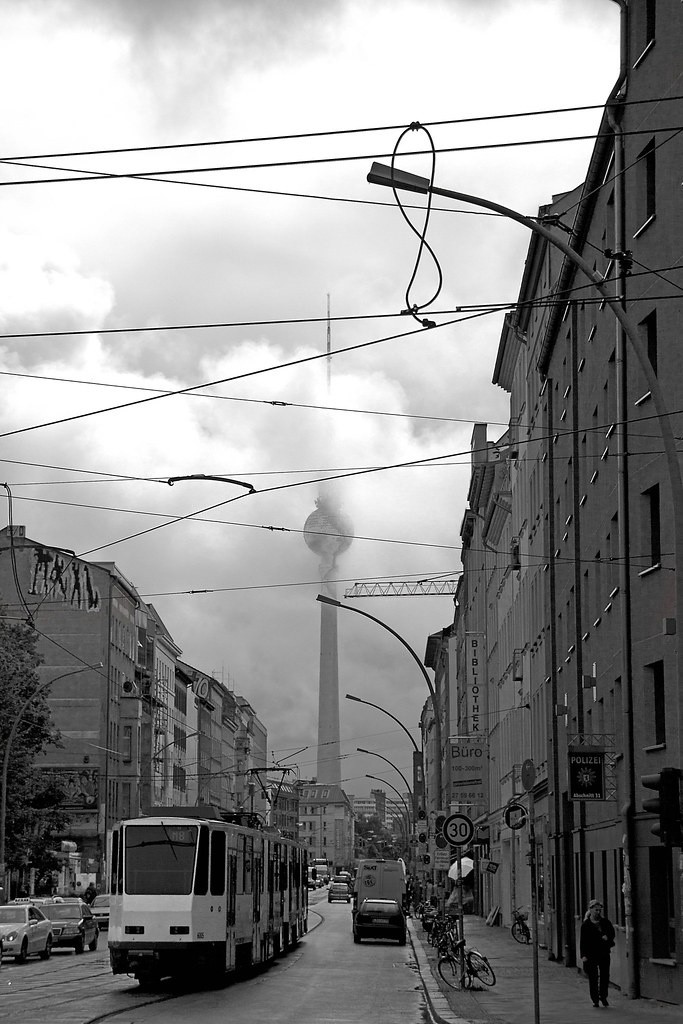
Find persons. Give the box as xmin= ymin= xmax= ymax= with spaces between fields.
xmin=580 ymin=900 xmax=615 ymax=1006
xmin=86 ymin=882 xmax=97 ymax=904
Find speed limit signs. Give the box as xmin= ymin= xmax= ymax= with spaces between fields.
xmin=443 ymin=813 xmax=473 ymax=847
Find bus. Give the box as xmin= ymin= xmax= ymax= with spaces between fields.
xmin=109 ymin=810 xmax=309 ymax=984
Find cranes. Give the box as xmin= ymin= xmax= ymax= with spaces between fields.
xmin=345 ymin=580 xmax=458 ymax=601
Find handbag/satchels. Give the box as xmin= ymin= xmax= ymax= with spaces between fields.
xmin=599 ymin=928 xmax=615 ymax=949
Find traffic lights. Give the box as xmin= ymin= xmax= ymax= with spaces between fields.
xmin=639 ymin=770 xmax=682 ymax=849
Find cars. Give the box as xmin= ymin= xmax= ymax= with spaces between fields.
xmin=331 ymin=875 xmax=348 ymax=885
xmin=41 ymin=902 xmax=98 ymax=954
xmin=354 ymin=899 xmax=407 ymax=948
xmin=327 ymin=881 xmax=350 ymax=905
xmin=307 ymin=867 xmax=316 ymax=891
xmin=90 ymin=896 xmax=111 ymax=930
xmin=0 ymin=906 xmax=51 ymax=960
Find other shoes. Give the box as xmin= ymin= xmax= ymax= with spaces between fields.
xmin=599 ymin=996 xmax=609 ymax=1006
xmin=593 ymin=1002 xmax=600 ymax=1007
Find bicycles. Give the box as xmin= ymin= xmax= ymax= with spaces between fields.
xmin=439 ymin=924 xmax=496 ymax=991
xmin=415 ymin=899 xmax=457 ymax=949
xmin=510 ymin=907 xmax=533 ymax=946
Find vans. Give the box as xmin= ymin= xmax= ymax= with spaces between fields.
xmin=356 ymin=859 xmax=406 ymax=915
xmin=314 ymin=865 xmax=329 ymax=886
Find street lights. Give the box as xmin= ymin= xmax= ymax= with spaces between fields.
xmin=364 ymin=774 xmax=411 ymax=848
xmin=193 ymin=763 xmax=244 ymax=810
xmin=317 ymin=594 xmax=444 ymax=921
xmin=371 ymin=800 xmax=404 ymax=836
xmin=363 ymin=773 xmax=410 ymax=841
xmin=357 ymin=747 xmax=416 ymax=842
xmin=368 ymin=791 xmax=408 ymax=838
xmin=135 ymin=731 xmax=203 ymax=819
xmin=346 ymin=693 xmax=426 ymax=826
xmin=365 ymin=161 xmax=681 ymax=819
xmin=2 ymin=663 xmax=105 ymax=893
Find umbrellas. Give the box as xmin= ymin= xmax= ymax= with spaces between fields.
xmin=448 ymin=857 xmax=474 ymax=880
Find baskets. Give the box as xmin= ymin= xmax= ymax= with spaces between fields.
xmin=514 ymin=912 xmax=528 ymax=921
